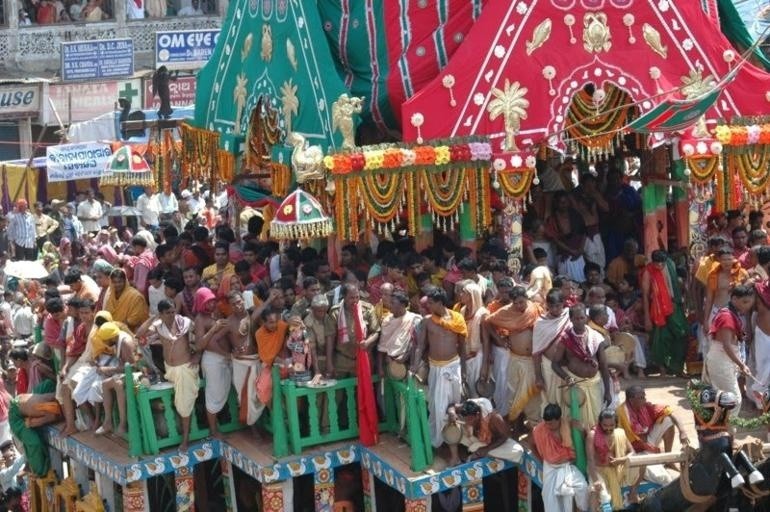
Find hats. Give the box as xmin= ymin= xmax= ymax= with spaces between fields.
xmin=50 ymin=198 xmax=65 ymax=206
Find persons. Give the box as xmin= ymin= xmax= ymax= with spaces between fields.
xmin=332 ymin=93 xmax=362 ymax=150
xmin=0 ymin=160 xmax=769 ymax=512
xmin=152 ymin=66 xmax=179 ymax=118
xmin=18 ymin=0 xmax=203 ymax=27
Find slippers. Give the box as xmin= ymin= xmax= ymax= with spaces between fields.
xmin=93 ymin=425 xmax=112 ymax=436
